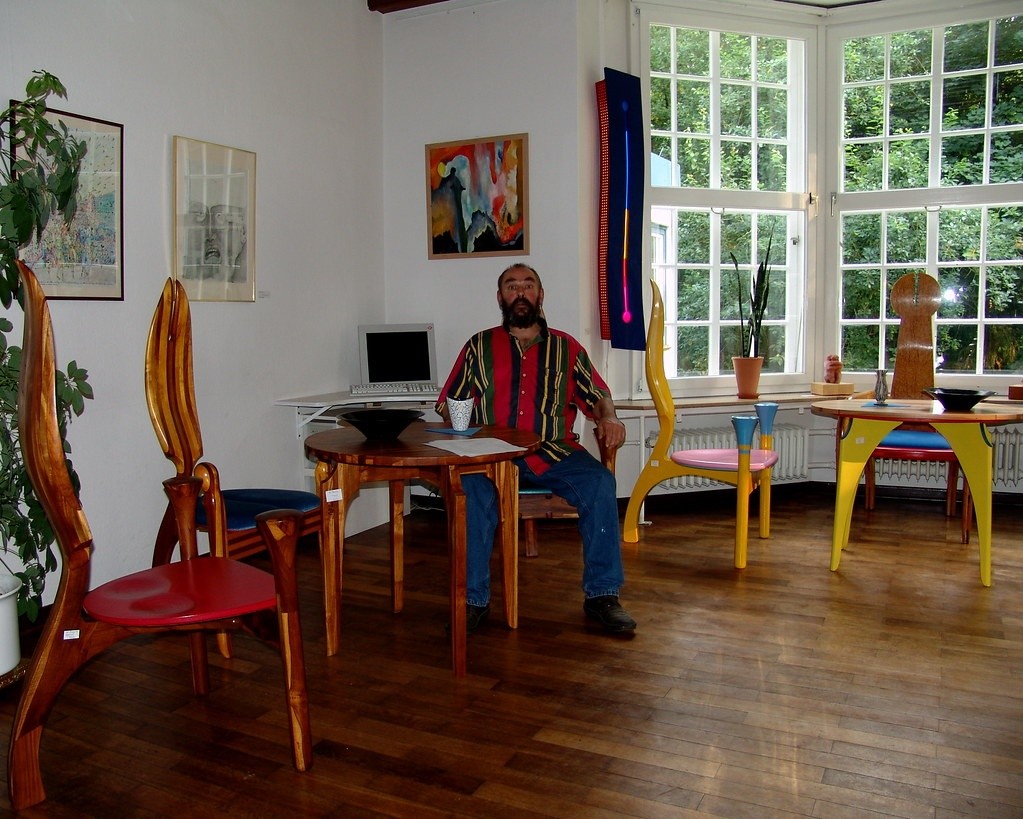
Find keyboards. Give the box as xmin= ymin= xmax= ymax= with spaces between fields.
xmin=349 ymin=384 xmax=440 ymax=395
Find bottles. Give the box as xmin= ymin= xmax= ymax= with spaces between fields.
xmin=874 ymin=369 xmax=889 ymax=403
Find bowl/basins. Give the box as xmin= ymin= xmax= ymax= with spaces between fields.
xmin=923 ymin=387 xmax=999 ymax=412
xmin=337 ymin=408 xmax=425 ymax=445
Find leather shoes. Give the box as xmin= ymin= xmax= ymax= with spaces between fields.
xmin=582 ymin=596 xmax=636 ymax=633
xmin=445 ymin=603 xmax=491 ymax=637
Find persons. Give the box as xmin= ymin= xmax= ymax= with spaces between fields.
xmin=434 ymin=263 xmax=638 ymax=640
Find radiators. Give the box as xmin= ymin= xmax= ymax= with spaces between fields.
xmin=874 ymin=426 xmax=1023 ymax=487
xmin=645 ymin=422 xmax=808 ymax=490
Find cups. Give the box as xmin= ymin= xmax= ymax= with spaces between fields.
xmin=447 ymin=394 xmax=475 ymax=431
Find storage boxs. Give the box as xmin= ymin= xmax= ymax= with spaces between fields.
xmin=810 ymin=382 xmax=854 ymax=396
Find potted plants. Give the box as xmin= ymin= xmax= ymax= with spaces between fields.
xmin=729 ymin=220 xmax=776 ymax=399
xmin=0 ymin=68 xmax=94 ymax=675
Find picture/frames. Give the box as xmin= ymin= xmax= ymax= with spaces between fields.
xmin=9 ymin=99 xmax=126 ymax=302
xmin=172 ymin=135 xmax=258 ymax=302
xmin=425 ymin=133 xmax=530 ymax=259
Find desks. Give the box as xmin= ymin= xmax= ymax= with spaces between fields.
xmin=303 ymin=422 xmax=544 ymax=687
xmin=277 ymin=390 xmax=440 ymax=493
xmin=811 ymin=399 xmax=1023 ymax=587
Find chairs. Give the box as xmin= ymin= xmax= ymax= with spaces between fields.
xmin=842 ymin=272 xmax=973 ymax=544
xmin=520 ymin=428 xmax=617 ymax=556
xmin=7 ymin=258 xmax=311 ymax=819
xmin=148 ymin=278 xmax=320 ymax=656
xmin=623 ymin=279 xmax=781 ymax=569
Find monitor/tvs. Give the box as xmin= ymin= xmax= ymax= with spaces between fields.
xmin=358 ymin=323 xmax=438 ymax=385
